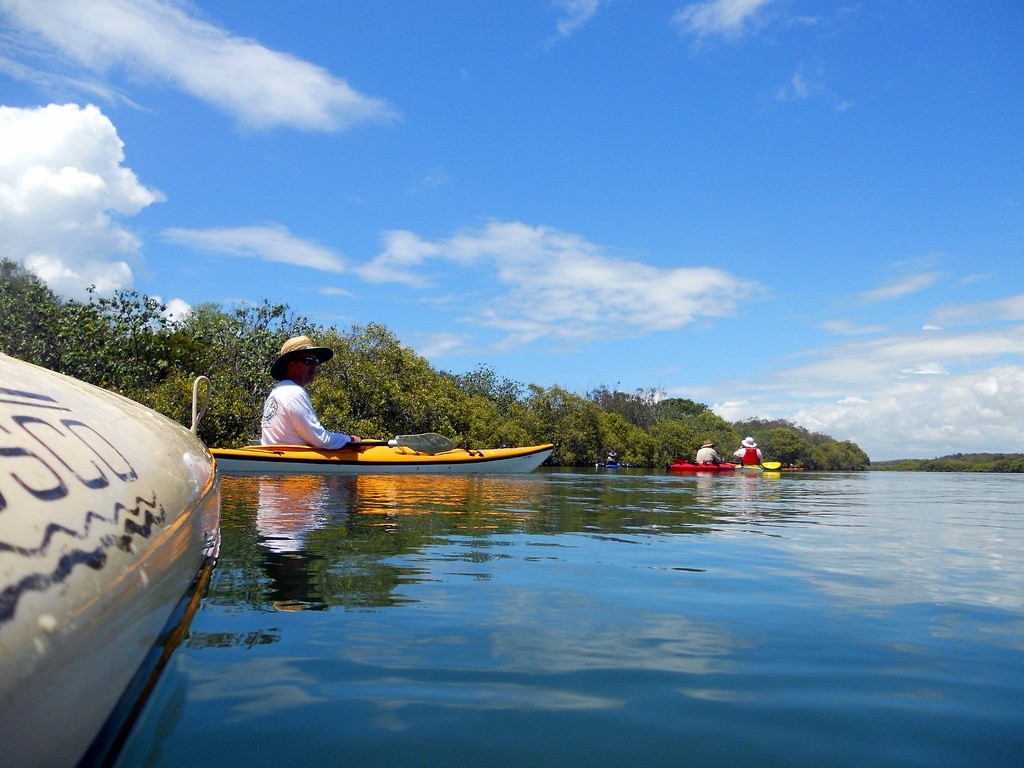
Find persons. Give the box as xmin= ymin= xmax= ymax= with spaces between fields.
xmin=260 ymin=334 xmax=362 ymax=450
xmin=789 ymin=463 xmax=795 ymax=468
xmin=734 ymin=436 xmax=763 ymax=465
xmin=607 ymin=451 xmax=618 ymax=465
xmin=696 ymin=438 xmax=727 ymax=465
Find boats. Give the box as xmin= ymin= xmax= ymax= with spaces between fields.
xmin=666 ymin=456 xmax=738 ymax=473
xmin=781 ymin=467 xmax=805 ymax=471
xmin=205 ymin=439 xmax=556 ymax=472
xmin=594 ymin=462 xmax=621 ymax=468
xmin=734 ymin=463 xmax=762 ymax=470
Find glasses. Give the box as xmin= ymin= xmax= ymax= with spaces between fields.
xmin=296 ymin=355 xmax=321 ymax=366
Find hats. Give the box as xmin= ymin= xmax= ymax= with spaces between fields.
xmin=269 ymin=335 xmax=334 ymax=382
xmin=741 ymin=437 xmax=757 ymax=448
xmin=701 ymin=438 xmax=716 ymax=448
xmin=608 ymin=450 xmax=617 ymax=456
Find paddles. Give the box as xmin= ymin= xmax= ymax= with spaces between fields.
xmin=346 ymin=432 xmax=456 ymax=453
